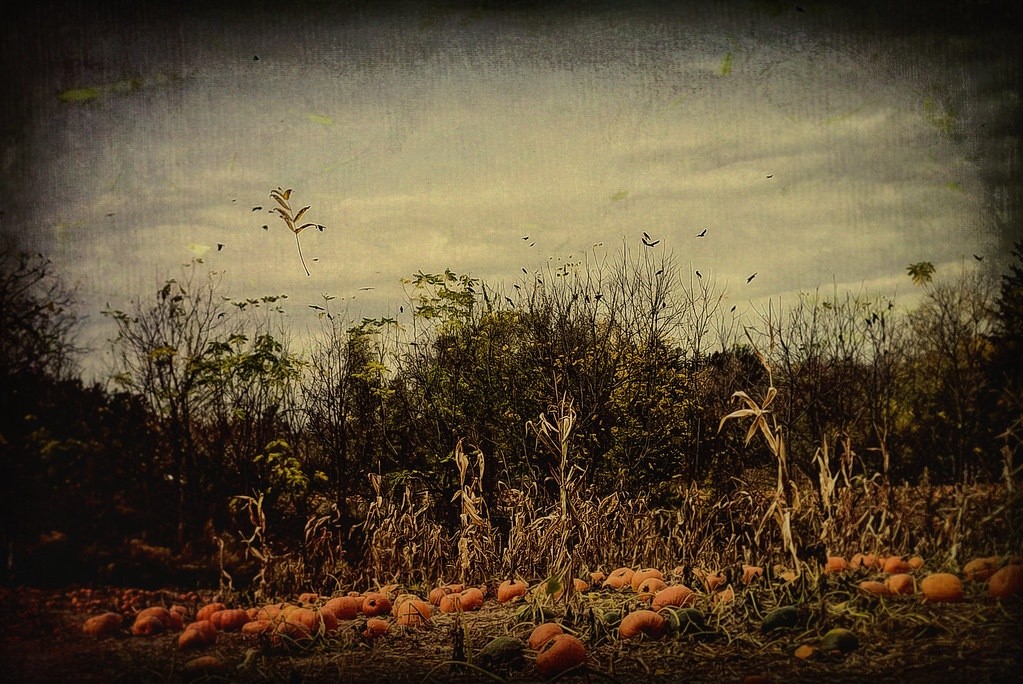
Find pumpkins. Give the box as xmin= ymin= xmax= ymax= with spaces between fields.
xmin=494 ymin=552 xmax=1023 ymax=675
xmin=0 ymin=584 xmax=485 ymax=684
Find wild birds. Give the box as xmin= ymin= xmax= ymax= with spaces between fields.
xmin=106 ymin=174 xmax=759 ymax=336
